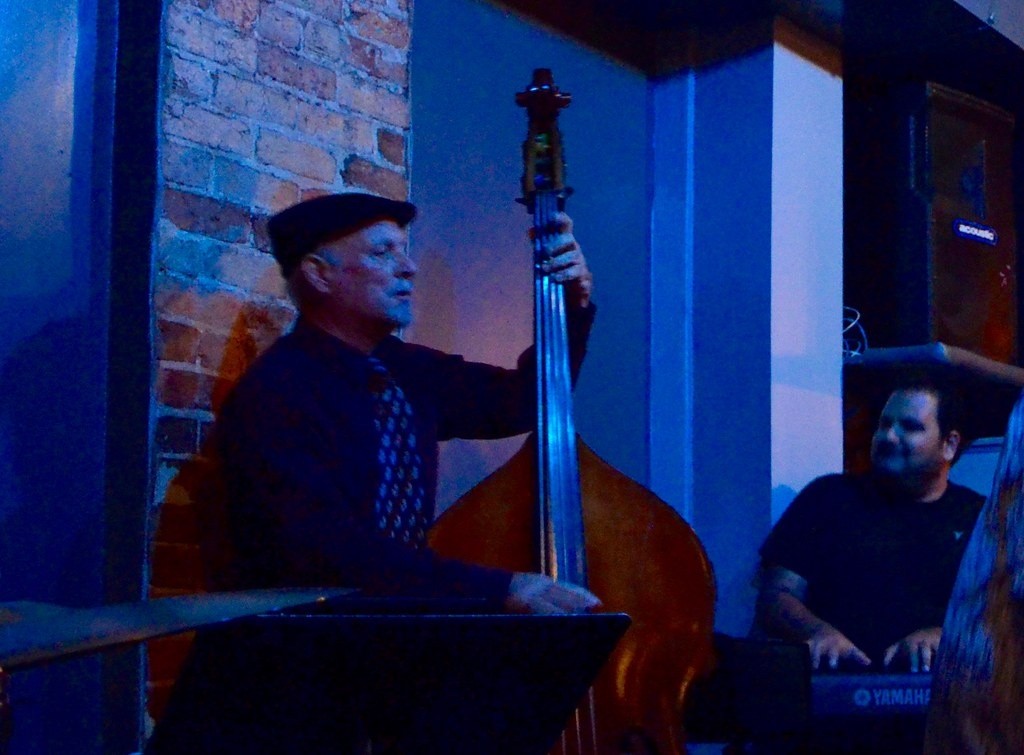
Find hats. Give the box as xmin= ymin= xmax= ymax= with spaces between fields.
xmin=267 ymin=193 xmax=417 ymax=268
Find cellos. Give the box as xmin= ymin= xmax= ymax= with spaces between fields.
xmin=423 ymin=68 xmax=718 ymax=755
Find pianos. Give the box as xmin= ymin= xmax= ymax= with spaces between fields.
xmin=729 ymin=617 xmax=935 ymax=734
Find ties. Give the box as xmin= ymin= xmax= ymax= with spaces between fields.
xmin=369 ymin=357 xmax=429 ymax=552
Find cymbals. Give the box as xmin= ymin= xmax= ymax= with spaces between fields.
xmin=0 ymin=587 xmax=357 ymax=670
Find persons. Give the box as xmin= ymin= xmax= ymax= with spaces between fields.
xmin=133 ymin=187 xmax=602 ymax=754
xmin=739 ymin=371 xmax=991 ymax=750
